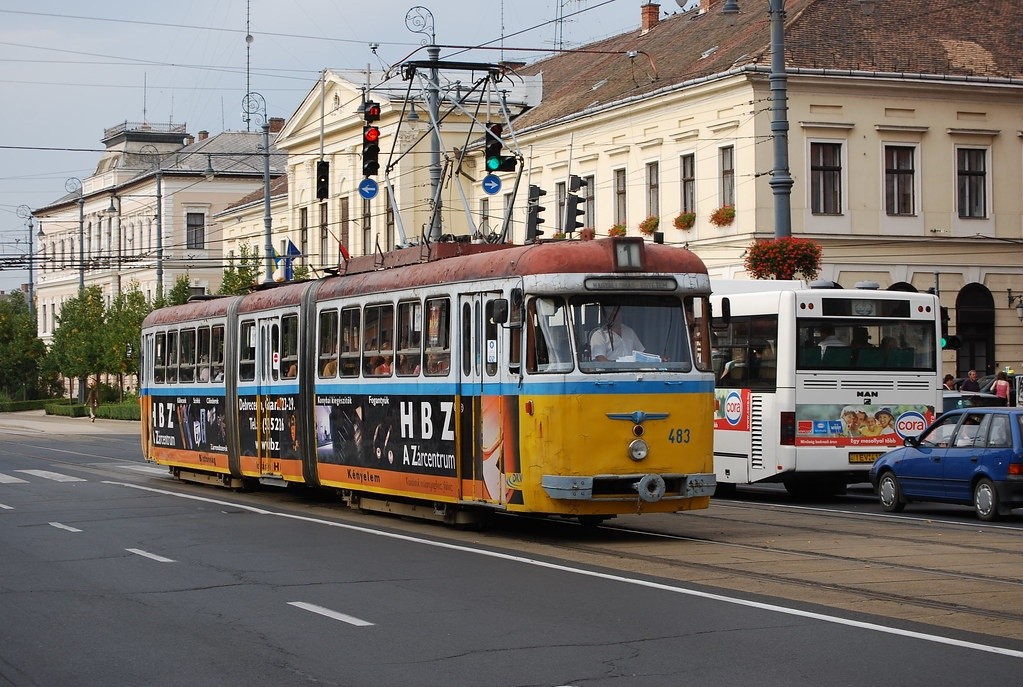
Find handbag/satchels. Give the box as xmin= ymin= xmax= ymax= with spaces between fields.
xmin=94 ymin=399 xmax=97 ymax=406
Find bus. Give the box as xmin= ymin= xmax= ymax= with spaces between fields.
xmin=536 ymin=278 xmax=944 ymax=506
xmin=131 ymin=58 xmax=717 ymax=528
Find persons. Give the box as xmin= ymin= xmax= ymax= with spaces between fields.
xmin=322 ymin=335 xmax=447 ymax=376
xmin=590 ymin=300 xmax=671 ymax=370
xmin=943 ymin=374 xmax=954 ymax=390
xmin=84 ymin=385 xmax=98 ymax=422
xmin=125 ymin=387 xmax=130 ymax=397
xmin=697 ymin=333 xmax=774 ymax=378
xmin=288 ymin=363 xmax=297 ymax=377
xmin=155 ymin=354 xmax=223 ymax=382
xmin=836 ymin=407 xmax=895 ymax=437
xmin=990 ymin=372 xmax=1010 ymax=407
xmin=939 ymin=419 xmax=977 ymax=447
xmin=961 ymin=370 xmax=980 ymax=392
xmin=817 ymin=325 xmax=899 ymax=361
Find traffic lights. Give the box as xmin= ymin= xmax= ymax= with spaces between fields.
xmin=938 ymin=305 xmax=952 ymax=350
xmin=361 ymin=101 xmax=381 ymax=178
xmin=485 ymin=122 xmax=520 ymax=173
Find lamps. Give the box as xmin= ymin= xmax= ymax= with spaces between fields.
xmin=1007 ymin=288 xmax=1023 ymax=322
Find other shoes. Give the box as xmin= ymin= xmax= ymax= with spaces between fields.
xmin=91 ymin=416 xmax=95 ymax=423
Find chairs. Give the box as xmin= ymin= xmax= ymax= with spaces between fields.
xmin=709 ymin=356 xmax=775 ymax=387
xmin=798 ymin=345 xmax=914 ymax=371
xmin=589 ymin=327 xmax=601 ymax=345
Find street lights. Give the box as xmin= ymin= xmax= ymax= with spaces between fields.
xmin=106 ymin=141 xmax=165 ymax=308
xmin=718 ymin=0 xmax=798 ymax=282
xmin=33 ymin=175 xmax=88 ymax=406
xmin=400 ymin=7 xmax=513 ymax=244
xmin=204 ymin=88 xmax=279 ymax=284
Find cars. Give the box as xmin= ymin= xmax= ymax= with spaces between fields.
xmin=940 ymin=388 xmax=1010 ymax=446
xmin=867 ymin=404 xmax=1023 ymax=519
xmin=976 ymin=373 xmax=1023 ymax=408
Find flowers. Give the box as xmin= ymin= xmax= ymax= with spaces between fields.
xmin=738 ymin=236 xmax=823 ymax=286
xmin=709 ymin=206 xmax=737 ymax=230
xmin=639 ymin=215 xmax=659 ymax=238
xmin=672 ymin=210 xmax=696 ymax=233
xmin=607 ymin=223 xmax=626 ymax=237
xmin=552 ymin=232 xmax=566 ymax=239
